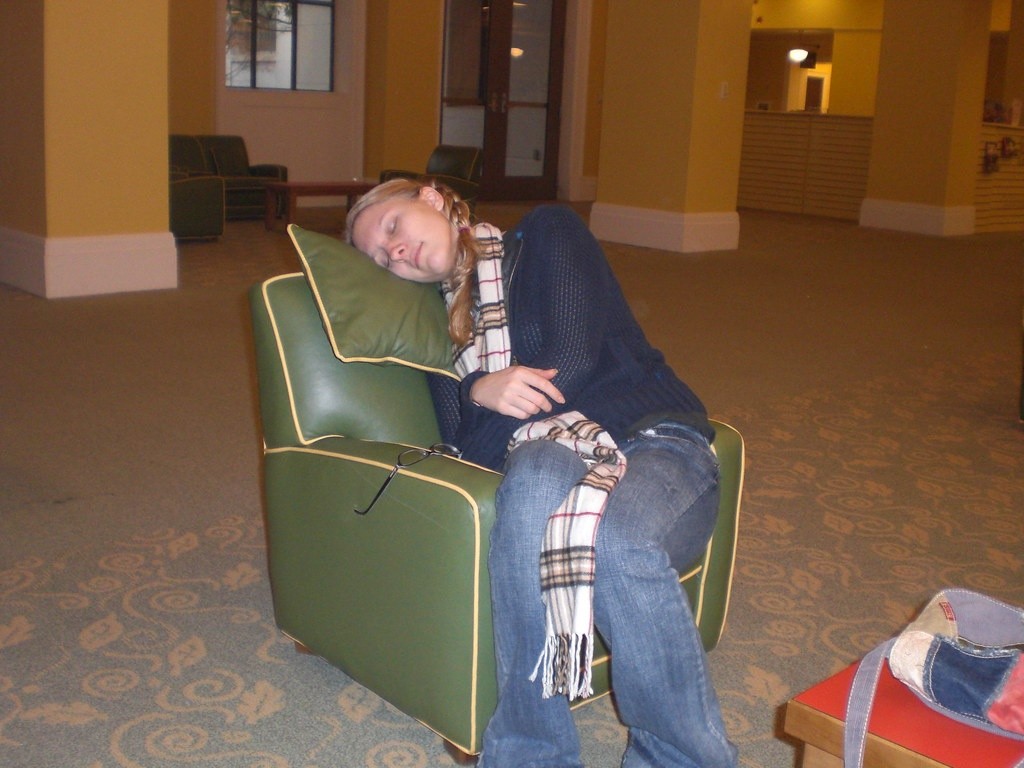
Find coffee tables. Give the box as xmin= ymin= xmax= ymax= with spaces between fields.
xmin=261 ymin=181 xmax=380 ymax=235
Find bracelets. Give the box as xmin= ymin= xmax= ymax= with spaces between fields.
xmin=468 ymin=386 xmax=483 ymax=407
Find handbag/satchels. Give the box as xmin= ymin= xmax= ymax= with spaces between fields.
xmin=887 ymin=588 xmax=1024 ymax=744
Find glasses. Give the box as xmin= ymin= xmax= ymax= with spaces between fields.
xmin=353 ymin=442 xmax=465 ymax=515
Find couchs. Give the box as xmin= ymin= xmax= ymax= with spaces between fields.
xmin=247 ymin=272 xmax=745 ymax=763
xmin=169 ymin=134 xmax=288 ymax=241
xmin=380 ymin=145 xmax=485 ymax=225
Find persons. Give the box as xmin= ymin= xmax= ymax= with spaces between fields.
xmin=344 ymin=173 xmax=737 ymax=768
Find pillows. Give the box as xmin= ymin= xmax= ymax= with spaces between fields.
xmin=286 ymin=224 xmax=460 ymax=381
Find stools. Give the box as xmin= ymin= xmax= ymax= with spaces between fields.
xmin=783 ymin=656 xmax=1024 ymax=768
